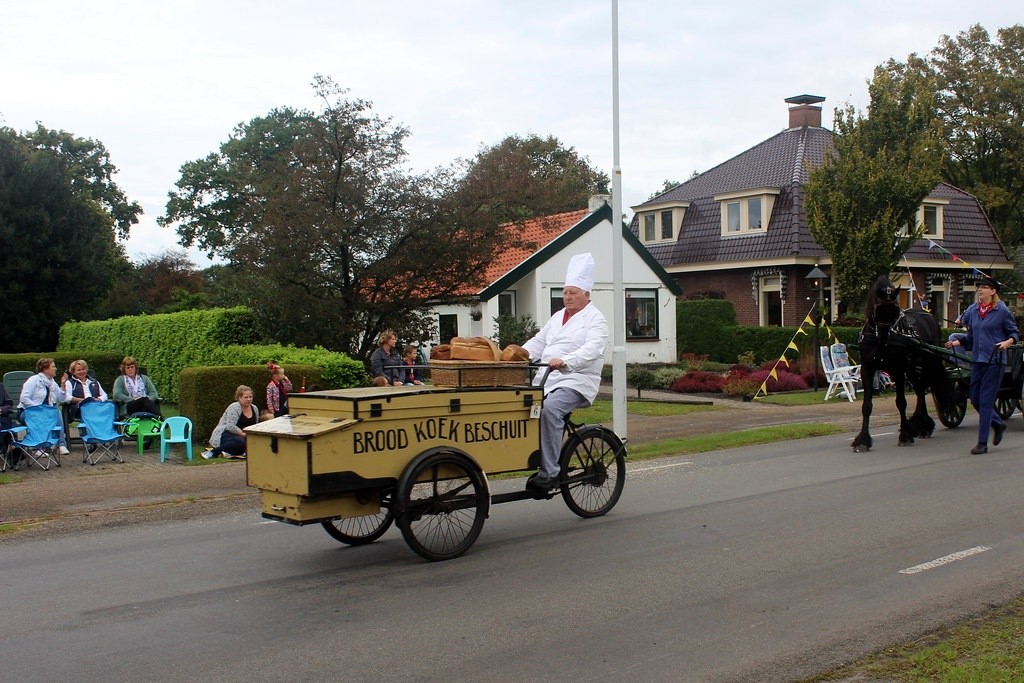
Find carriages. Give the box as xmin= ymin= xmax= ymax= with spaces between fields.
xmin=851 ymin=274 xmax=1024 ymax=455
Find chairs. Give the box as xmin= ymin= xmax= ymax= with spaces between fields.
xmin=120 ymin=397 xmax=163 ymax=444
xmin=131 ymin=415 xmax=166 ymax=455
xmin=159 ymin=416 xmax=192 ymax=463
xmin=3 ymin=371 xmax=35 ymax=425
xmin=818 ymin=343 xmax=893 ymax=403
xmin=81 ymin=400 xmax=130 ymax=465
xmin=0 ymin=404 xmax=61 ymax=471
xmin=63 ymin=409 xmax=117 ymax=452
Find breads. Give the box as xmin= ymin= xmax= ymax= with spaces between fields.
xmin=429 ymin=335 xmax=528 ymax=362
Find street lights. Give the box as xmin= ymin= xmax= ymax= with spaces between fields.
xmin=805 ymin=264 xmax=829 ymax=392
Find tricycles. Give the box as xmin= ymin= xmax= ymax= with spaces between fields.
xmin=244 ymin=359 xmax=629 ymax=562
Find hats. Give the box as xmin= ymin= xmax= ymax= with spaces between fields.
xmin=564 ymin=253 xmax=596 ymax=292
xmin=975 ymin=277 xmax=1000 ymax=294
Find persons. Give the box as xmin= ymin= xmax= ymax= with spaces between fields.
xmin=16 ymin=357 xmax=85 ymax=457
xmin=369 ymin=330 xmax=424 ymax=387
xmin=60 ymin=359 xmax=119 ymax=453
xmin=522 ymin=253 xmax=608 ymax=489
xmin=944 ymin=277 xmax=1019 ymax=453
xmin=265 ymin=364 xmax=294 ymax=419
xmin=113 ymin=357 xmax=168 ymax=450
xmin=0 ymin=384 xmax=26 ymax=464
xmin=200 ymin=385 xmax=260 ymax=460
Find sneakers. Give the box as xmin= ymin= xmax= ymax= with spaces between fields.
xmin=529 ymin=475 xmax=560 ymax=489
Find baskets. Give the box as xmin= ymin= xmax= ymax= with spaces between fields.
xmin=427 ymin=360 xmax=530 ymax=389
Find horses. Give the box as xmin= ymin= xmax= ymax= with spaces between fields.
xmin=849 ymin=273 xmax=943 ymax=453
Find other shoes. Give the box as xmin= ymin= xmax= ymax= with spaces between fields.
xmin=32 ymin=449 xmax=50 ymax=458
xmin=971 ymin=442 xmax=987 ymax=454
xmin=201 ymin=450 xmax=213 ymax=459
xmin=222 ymin=450 xmax=231 ymax=458
xmin=993 ymin=421 xmax=1007 ymax=446
xmin=944 ymin=366 xmax=961 ymax=371
xmin=56 ymin=445 xmax=69 ymax=455
